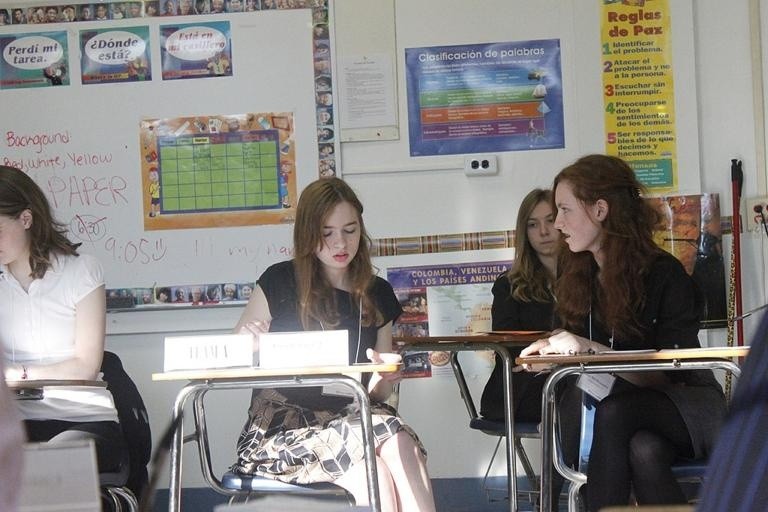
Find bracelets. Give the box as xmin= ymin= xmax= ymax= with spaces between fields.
xmin=20 ymin=363 xmax=27 ymax=381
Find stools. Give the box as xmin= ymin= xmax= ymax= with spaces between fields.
xmin=568 ymin=456 xmax=710 ymax=511
xmin=222 ymin=472 xmax=355 ymax=512
xmin=99 ymin=457 xmax=140 ymax=511
xmin=470 ymin=415 xmax=543 ymax=503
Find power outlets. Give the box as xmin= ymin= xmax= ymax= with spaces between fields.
xmin=745 ymin=197 xmax=768 ymax=231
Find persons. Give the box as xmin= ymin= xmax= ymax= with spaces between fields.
xmin=232 ymin=176 xmax=437 ymax=512
xmin=1 ymin=1 xmax=312 ymax=24
xmin=480 ymin=189 xmax=583 ymax=512
xmin=156 ymin=283 xmax=254 ymax=303
xmin=313 ymin=0 xmax=337 ymax=178
xmin=517 ymin=155 xmax=732 ymax=512
xmin=0 ymin=165 xmax=129 ymax=490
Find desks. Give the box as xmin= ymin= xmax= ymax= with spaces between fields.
xmin=8 ymin=379 xmax=107 ymax=401
xmin=152 ymin=363 xmax=400 ymax=512
xmin=392 ymin=333 xmax=555 ymax=512
xmin=515 ymin=346 xmax=751 ymax=512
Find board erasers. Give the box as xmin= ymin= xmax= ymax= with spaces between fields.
xmin=106 ymin=296 xmax=136 ymax=308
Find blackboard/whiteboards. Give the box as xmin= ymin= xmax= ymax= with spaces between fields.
xmin=0 ymin=0 xmax=343 ymax=312
